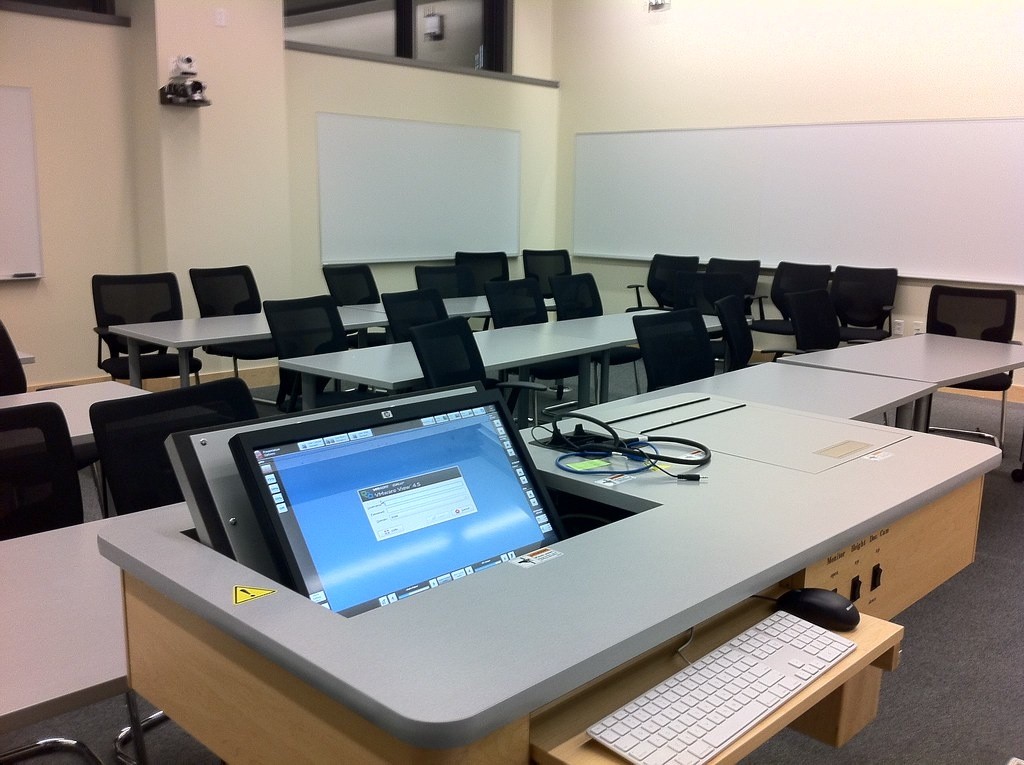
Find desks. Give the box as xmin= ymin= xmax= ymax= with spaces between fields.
xmin=109 ymin=295 xmax=754 ymax=393
xmin=279 ymin=308 xmax=752 ymax=419
xmin=0 ymin=501 xmax=194 ymax=765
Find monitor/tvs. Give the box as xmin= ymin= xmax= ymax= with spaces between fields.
xmin=227 ymin=386 xmax=571 ymax=620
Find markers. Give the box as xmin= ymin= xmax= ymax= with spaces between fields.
xmin=12 ymin=272 xmax=36 ymax=278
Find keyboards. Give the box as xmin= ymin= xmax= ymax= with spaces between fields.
xmin=586 ymin=611 xmax=859 ymax=765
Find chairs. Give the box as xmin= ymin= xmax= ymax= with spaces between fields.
xmin=564 ymin=325 xmax=1023 ymax=470
xmin=410 ymin=318 xmax=546 ymax=426
xmin=89 ymin=376 xmax=259 ymax=519
xmin=626 ymin=254 xmax=1016 ymax=456
xmin=0 ymin=402 xmax=84 ymax=541
xmin=713 ymin=294 xmax=805 ymax=373
xmin=0 ymin=379 xmax=150 ymax=520
xmin=92 ymin=249 xmax=648 ymax=418
xmin=631 ymin=307 xmax=716 ymax=390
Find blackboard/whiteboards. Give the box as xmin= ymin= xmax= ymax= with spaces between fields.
xmin=313 ymin=109 xmax=524 ymax=269
xmin=570 ymin=114 xmax=1024 ymax=288
xmin=0 ymin=84 xmax=45 ymax=282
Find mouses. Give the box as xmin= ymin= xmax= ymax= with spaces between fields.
xmin=775 ymin=587 xmax=861 ymax=630
